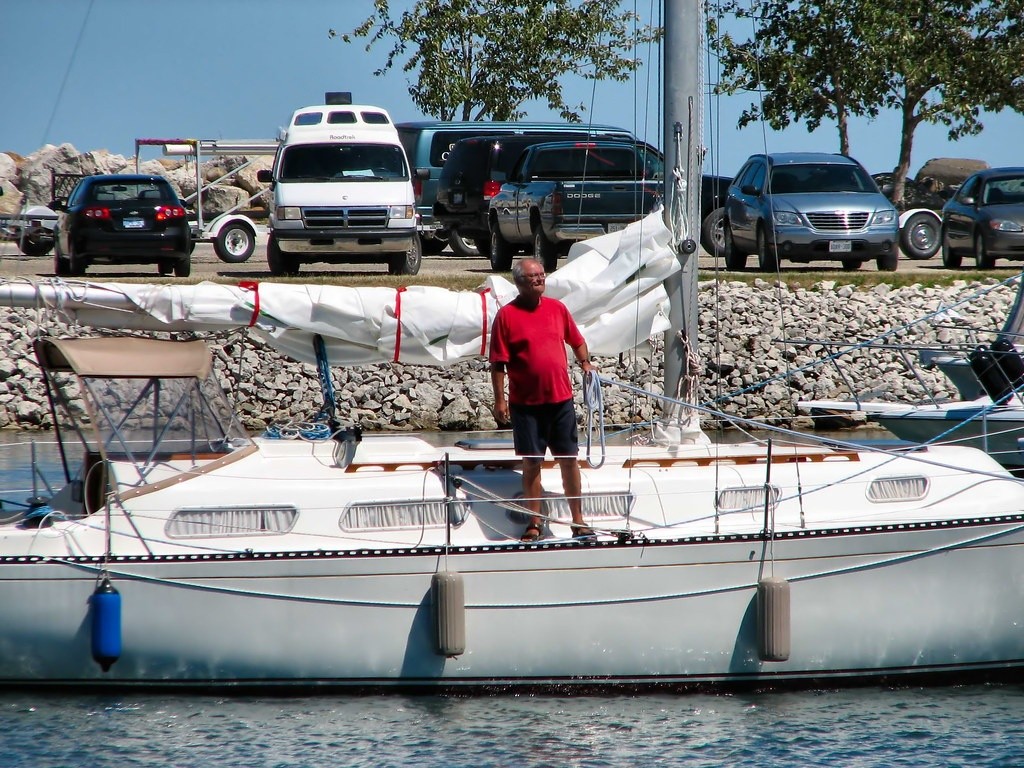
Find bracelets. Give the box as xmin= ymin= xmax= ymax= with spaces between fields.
xmin=580 ymin=359 xmax=591 ymax=364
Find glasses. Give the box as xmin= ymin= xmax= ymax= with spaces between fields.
xmin=518 ymin=272 xmax=547 ymax=281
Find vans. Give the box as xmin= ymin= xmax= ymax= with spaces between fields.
xmin=393 ymin=121 xmax=645 ymax=258
xmin=258 ymin=92 xmax=433 ymax=279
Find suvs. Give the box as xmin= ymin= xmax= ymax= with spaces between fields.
xmin=433 ymin=134 xmax=738 ymax=261
xmin=723 ymin=151 xmax=899 ymax=272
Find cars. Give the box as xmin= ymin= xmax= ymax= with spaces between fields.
xmin=47 ymin=174 xmax=191 ymax=279
xmin=870 ymin=172 xmax=959 ymax=260
xmin=941 ymin=166 xmax=1024 ymax=270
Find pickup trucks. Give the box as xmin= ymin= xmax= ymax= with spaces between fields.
xmin=488 ymin=140 xmax=664 ymax=275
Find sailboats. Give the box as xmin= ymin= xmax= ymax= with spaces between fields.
xmin=1 ymin=0 xmax=1024 ymax=696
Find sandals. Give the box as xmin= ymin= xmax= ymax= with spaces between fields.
xmin=572 ymin=523 xmax=596 ymax=541
xmin=521 ymin=523 xmax=545 ymax=542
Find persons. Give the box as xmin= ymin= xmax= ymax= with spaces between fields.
xmin=488 ymin=257 xmax=597 ymax=541
